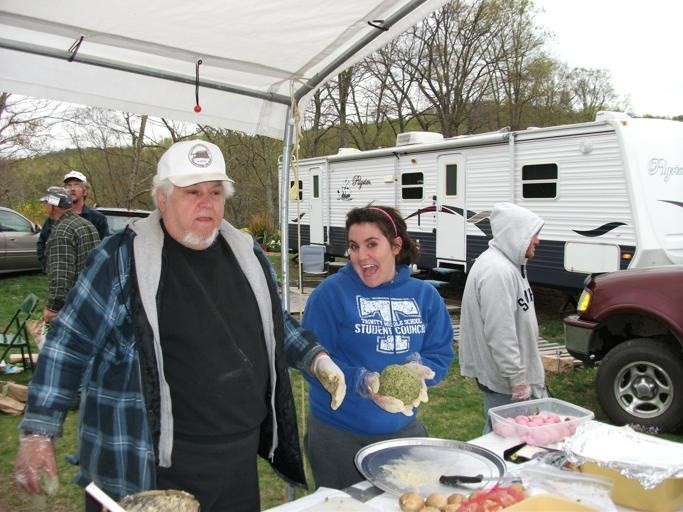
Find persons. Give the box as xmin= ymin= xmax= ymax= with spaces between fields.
xmin=10 ymin=132 xmax=349 ymax=512
xmin=33 ymin=170 xmax=109 ymax=275
xmin=456 ymin=200 xmax=549 ymax=435
xmin=302 ymin=202 xmax=456 ymax=490
xmin=38 ymin=185 xmax=103 ymax=410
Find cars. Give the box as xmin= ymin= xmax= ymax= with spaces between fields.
xmin=0 ymin=204 xmax=45 ymax=279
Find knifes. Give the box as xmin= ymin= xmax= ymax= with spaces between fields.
xmin=439 ymin=475 xmax=515 ymax=486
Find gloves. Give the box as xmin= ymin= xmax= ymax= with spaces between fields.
xmin=313 ymin=355 xmax=346 ymax=411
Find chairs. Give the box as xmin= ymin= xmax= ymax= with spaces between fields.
xmin=0 ymin=293 xmax=39 ymax=372
xmin=300 ymin=244 xmax=328 ymax=293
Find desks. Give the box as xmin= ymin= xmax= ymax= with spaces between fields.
xmin=262 ymin=411 xmax=683 ymax=512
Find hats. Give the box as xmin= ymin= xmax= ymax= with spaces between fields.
xmin=63 ymin=171 xmax=87 ymax=184
xmin=157 ymin=139 xmax=236 ymax=188
xmin=40 ymin=188 xmax=73 ymax=208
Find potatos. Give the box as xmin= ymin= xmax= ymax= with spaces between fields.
xmin=419 ymin=506 xmax=441 ymax=512
xmin=425 ymin=493 xmax=448 ymax=511
xmin=448 ymin=493 xmax=467 ymax=503
xmin=441 ymin=504 xmax=462 ymax=512
xmin=399 ymin=492 xmax=425 ymax=512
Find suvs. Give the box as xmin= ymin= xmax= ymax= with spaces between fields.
xmin=89 ymin=203 xmax=157 ymax=238
xmin=560 ymin=265 xmax=681 ymax=435
